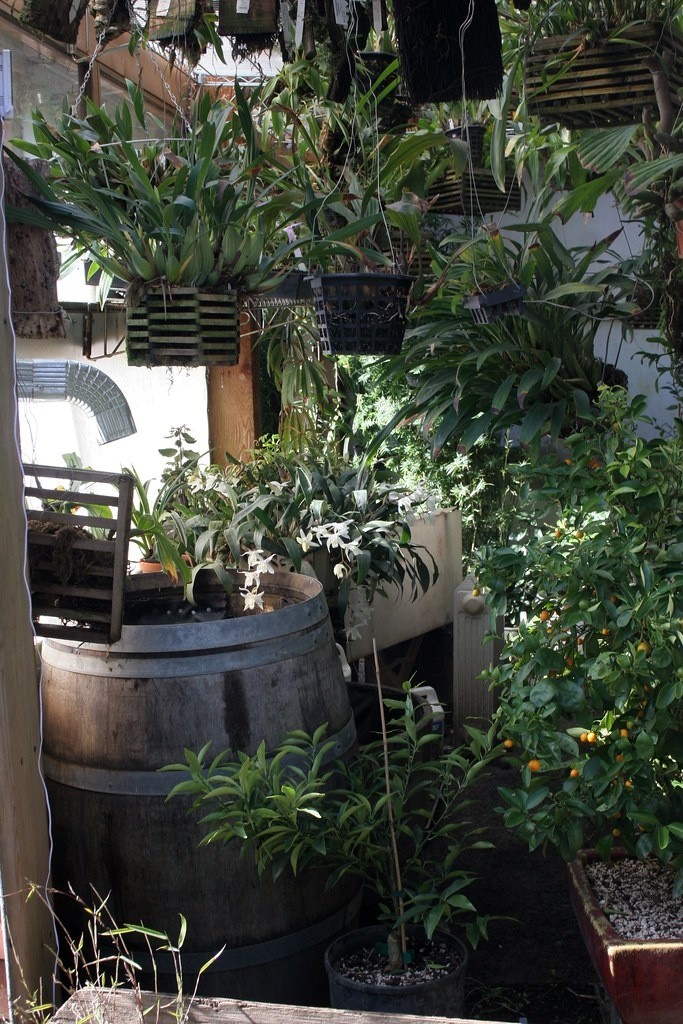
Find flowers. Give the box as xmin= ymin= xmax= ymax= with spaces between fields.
xmin=141 ymin=440 xmax=431 ymax=637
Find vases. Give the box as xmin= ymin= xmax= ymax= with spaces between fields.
xmin=301 ymin=506 xmax=464 ymax=662
xmin=568 ymin=848 xmax=683 ymax=1024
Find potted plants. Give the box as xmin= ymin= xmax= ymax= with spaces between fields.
xmin=517 ymin=1 xmax=683 ymax=131
xmin=445 ymin=229 xmax=543 ymax=327
xmin=12 ymin=90 xmax=288 ymax=369
xmin=294 ymin=79 xmax=421 ymax=356
xmin=422 ymin=101 xmax=535 ymax=220
xmin=618 ymin=219 xmax=683 ymax=329
xmin=356 ymin=25 xmax=413 ymax=136
xmin=168 ymin=675 xmax=515 ymax=1017
